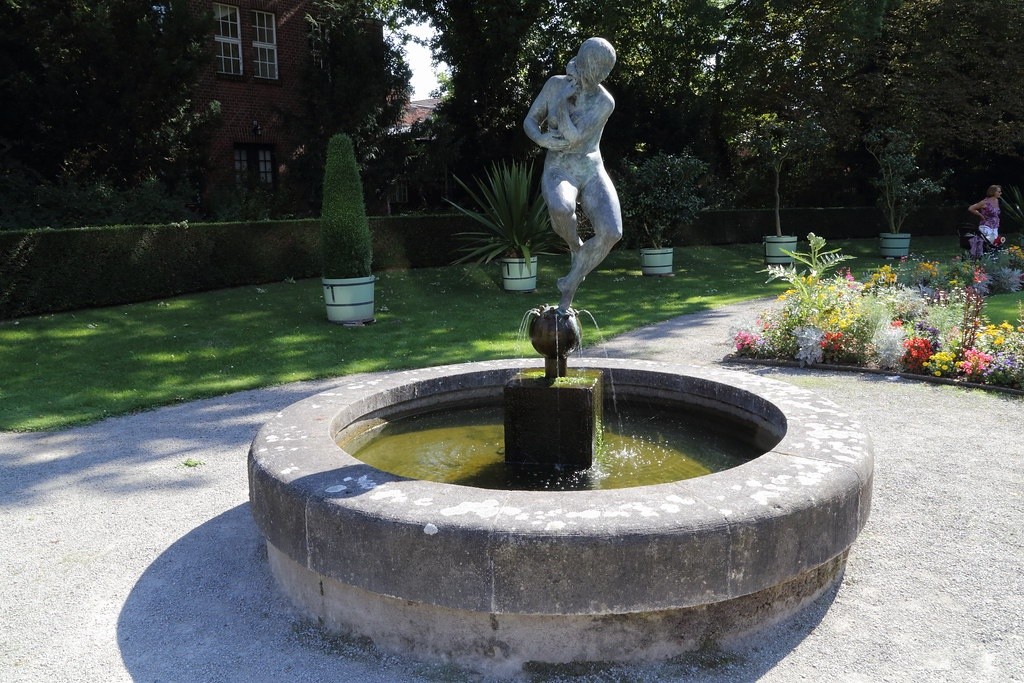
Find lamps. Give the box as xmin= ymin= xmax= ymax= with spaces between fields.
xmin=252 ymin=120 xmax=264 ymax=135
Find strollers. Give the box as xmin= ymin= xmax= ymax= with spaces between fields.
xmin=954 ymin=222 xmax=1007 ymax=261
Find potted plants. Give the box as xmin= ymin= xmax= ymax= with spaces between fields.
xmin=441 ymin=158 xmax=569 ymax=290
xmin=609 ymin=151 xmax=711 ymax=276
xmin=319 ymin=134 xmax=376 ymax=323
xmin=726 ymin=110 xmax=831 ymax=268
xmin=862 ymin=124 xmax=946 ymax=257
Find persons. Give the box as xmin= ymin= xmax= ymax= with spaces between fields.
xmin=968 ymin=185 xmax=1002 ymax=252
xmin=523 ymin=36 xmax=622 ymax=312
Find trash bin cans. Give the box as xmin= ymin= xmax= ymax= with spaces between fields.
xmin=957 ymin=224 xmax=978 ymax=249
